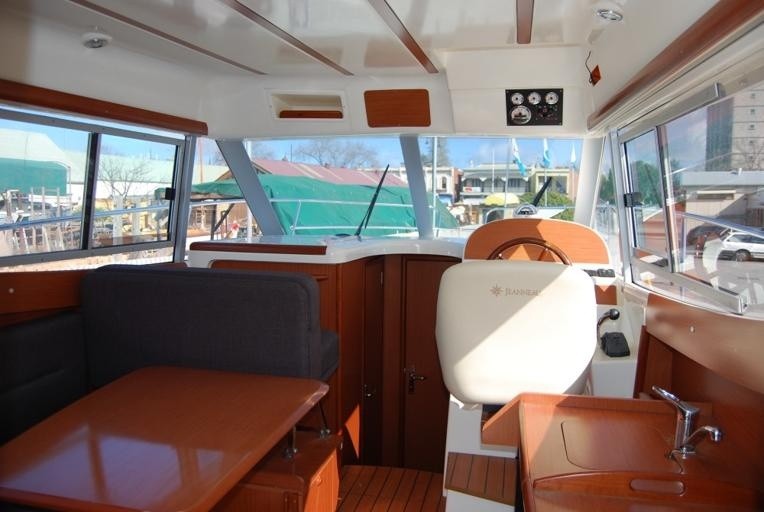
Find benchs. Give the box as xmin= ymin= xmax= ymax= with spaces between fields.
xmin=79 ymin=266 xmax=339 ymax=455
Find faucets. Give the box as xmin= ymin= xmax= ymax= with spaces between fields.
xmin=652 ymin=384 xmax=723 ymax=455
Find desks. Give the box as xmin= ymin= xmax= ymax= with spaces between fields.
xmin=0 ymin=364 xmax=333 ymax=512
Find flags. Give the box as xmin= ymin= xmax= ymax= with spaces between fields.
xmin=568 ymin=141 xmax=577 ymax=171
xmin=511 ymin=138 xmax=526 ymax=178
xmin=541 ymin=138 xmax=552 ymax=170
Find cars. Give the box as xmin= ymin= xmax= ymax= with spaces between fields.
xmin=695 ymin=223 xmax=763 ymax=261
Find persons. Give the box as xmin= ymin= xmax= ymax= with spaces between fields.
xmin=0 ymin=199 xmax=13 ymax=256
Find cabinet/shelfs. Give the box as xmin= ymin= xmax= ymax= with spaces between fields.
xmin=341 ymin=255 xmax=449 ymax=471
